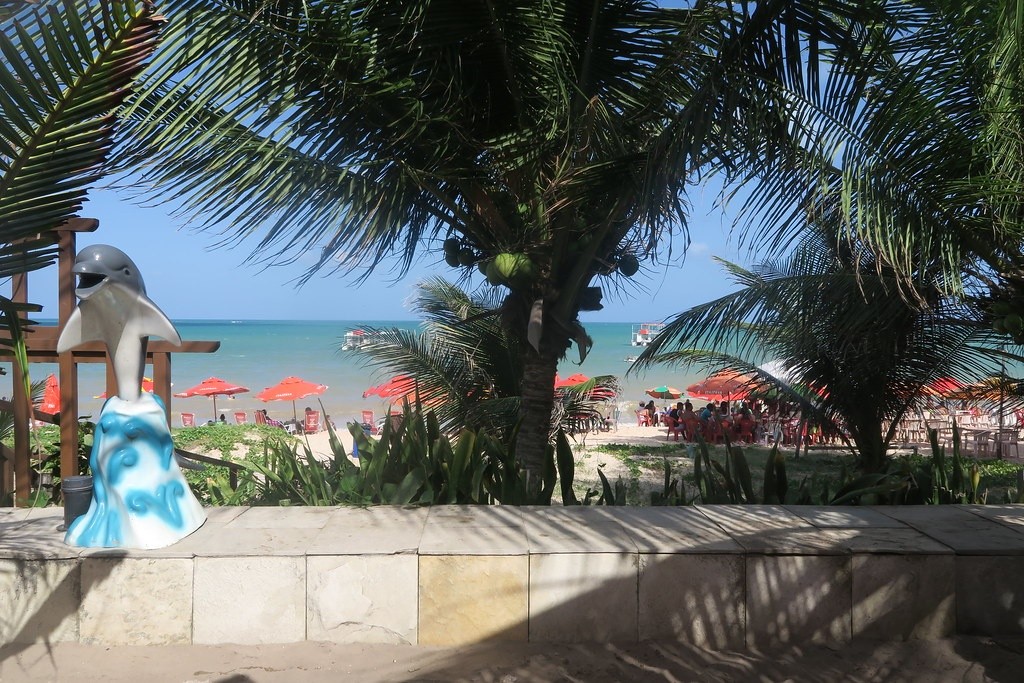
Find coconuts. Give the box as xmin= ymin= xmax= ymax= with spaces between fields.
xmin=993 ymin=301 xmax=1024 ymax=346
xmin=445 ymin=238 xmax=531 ymax=286
xmin=619 ymin=255 xmax=639 ymax=275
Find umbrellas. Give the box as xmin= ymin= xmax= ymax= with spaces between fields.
xmin=361 ymin=372 xmax=479 ymax=408
xmin=172 ymin=376 xmax=250 ymax=423
xmin=890 ymin=372 xmax=1024 ymax=421
xmin=93 ymin=376 xmax=174 ymax=399
xmin=645 ymin=384 xmax=684 ymax=399
xmin=554 ymin=372 xmax=590 ymax=386
xmin=38 ymin=373 xmax=61 ymax=415
xmin=755 ymin=353 xmax=839 ymax=396
xmin=686 ymin=367 xmax=772 ymax=419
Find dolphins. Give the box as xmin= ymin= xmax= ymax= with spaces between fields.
xmin=56 ymin=244 xmax=182 ymax=400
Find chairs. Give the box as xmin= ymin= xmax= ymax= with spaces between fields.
xmin=180 ymin=413 xmax=206 ymax=427
xmin=298 ymin=411 xmax=319 ymax=434
xmin=882 ymin=414 xmax=1021 ymax=460
xmin=634 ymin=409 xmax=666 ymax=427
xmin=216 ymin=421 xmax=232 ymax=427
xmin=664 ymin=410 xmax=850 ymax=445
xmin=362 ymin=411 xmax=403 ymax=434
xmin=255 ymin=410 xmax=296 ymax=435
xmin=234 ymin=412 xmax=248 ymax=425
xmin=599 ymin=405 xmax=620 ymax=433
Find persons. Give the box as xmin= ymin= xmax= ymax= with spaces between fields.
xmin=261 ymin=407 xmax=337 ymax=431
xmin=909 ymin=394 xmax=918 ymax=414
xmin=252 ymin=376 xmax=329 ymax=420
xmin=637 ymin=396 xmax=854 ymax=446
xmin=926 ymin=399 xmax=934 ymax=409
xmin=219 ymin=414 xmax=226 ymax=422
xmin=935 ymin=401 xmax=946 ymax=409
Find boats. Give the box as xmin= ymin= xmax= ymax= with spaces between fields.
xmin=631 ymin=322 xmax=668 ymax=347
xmin=342 ymin=329 xmax=405 ymax=352
